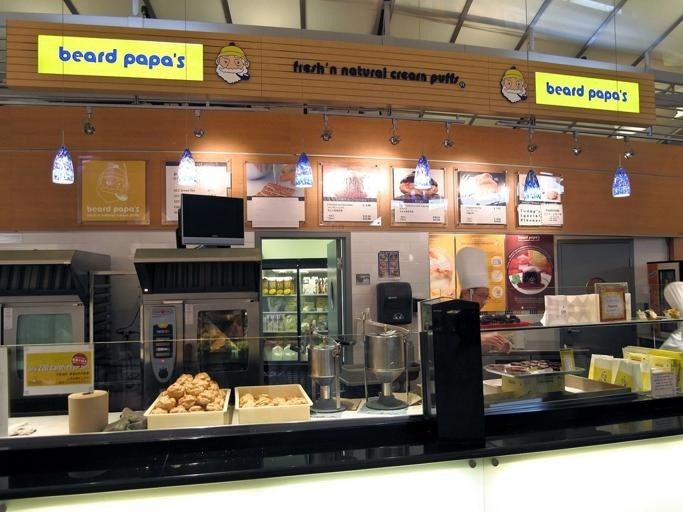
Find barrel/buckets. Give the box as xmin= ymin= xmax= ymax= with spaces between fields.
xmin=271 ymin=343 xmax=284 ymax=361
xmin=282 ymin=343 xmax=297 ymax=360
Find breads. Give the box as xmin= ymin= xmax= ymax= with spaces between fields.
xmin=464 ymin=173 xmax=497 ymax=196
xmin=239 ymin=392 xmax=307 ymax=408
xmin=153 ymin=373 xmax=225 ymax=413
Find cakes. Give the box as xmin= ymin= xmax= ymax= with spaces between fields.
xmin=400 ymin=170 xmax=439 ymax=196
xmin=487 ymin=359 xmax=561 ymax=375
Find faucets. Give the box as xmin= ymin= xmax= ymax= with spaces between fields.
xmin=585 ymin=277 xmax=606 ymax=288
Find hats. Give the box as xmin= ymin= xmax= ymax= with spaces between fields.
xmin=456 ymin=247 xmax=490 ymax=291
xmin=664 ymin=282 xmax=683 ymax=322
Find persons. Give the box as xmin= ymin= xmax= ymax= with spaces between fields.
xmin=658 ymin=318 xmax=683 ymax=352
xmin=457 ymin=285 xmax=513 ymax=354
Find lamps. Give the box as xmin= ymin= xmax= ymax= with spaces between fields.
xmin=51 ymin=0 xmax=75 ymax=187
xmin=292 ymin=0 xmax=313 ymax=189
xmin=411 ymin=0 xmax=432 ymax=189
xmin=177 ymin=1 xmax=198 ymax=188
xmin=609 ymin=0 xmax=632 ymax=199
xmin=519 ymin=0 xmax=540 ymax=202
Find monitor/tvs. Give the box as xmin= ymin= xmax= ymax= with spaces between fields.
xmin=181 ymin=193 xmax=244 ymax=248
